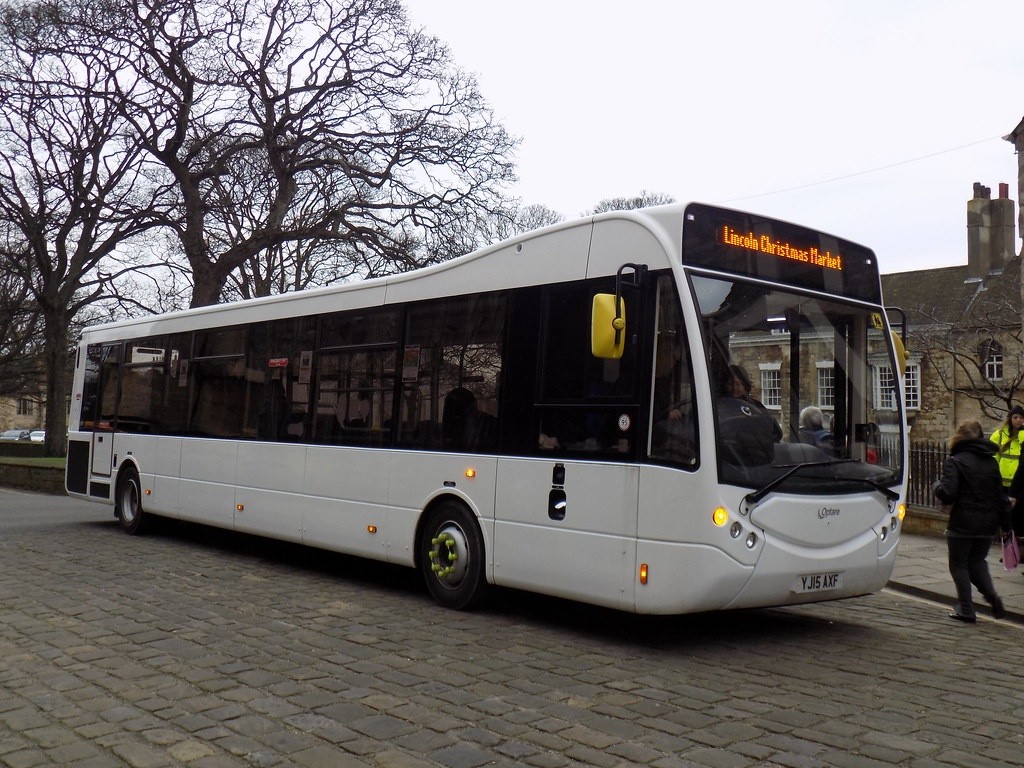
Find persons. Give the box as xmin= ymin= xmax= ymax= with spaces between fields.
xmin=933 ymin=420 xmax=1003 ymax=623
xmin=131 ymin=369 xmax=163 ymax=418
xmin=726 ymin=367 xmax=783 ymax=441
xmin=441 ymin=388 xmax=478 ymax=452
xmin=607 ymin=337 xmax=684 ymax=448
xmin=799 ymin=406 xmax=876 ymax=465
xmin=338 ymin=379 xmax=369 ymax=427
xmin=989 ymin=406 xmax=1024 ymax=576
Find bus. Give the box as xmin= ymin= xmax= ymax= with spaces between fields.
xmin=65 ymin=202 xmax=911 ymax=617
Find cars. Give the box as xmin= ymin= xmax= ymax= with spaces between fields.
xmin=0 ymin=429 xmax=45 ymax=442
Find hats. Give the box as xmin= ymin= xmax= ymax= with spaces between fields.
xmin=1007 ymin=405 xmax=1024 ymax=417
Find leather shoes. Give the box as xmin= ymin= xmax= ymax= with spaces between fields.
xmin=992 ymin=608 xmax=1003 ymax=618
xmin=949 ymin=612 xmax=976 ymax=623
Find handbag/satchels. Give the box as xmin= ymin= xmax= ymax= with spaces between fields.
xmin=1002 ymin=530 xmax=1021 ymax=570
